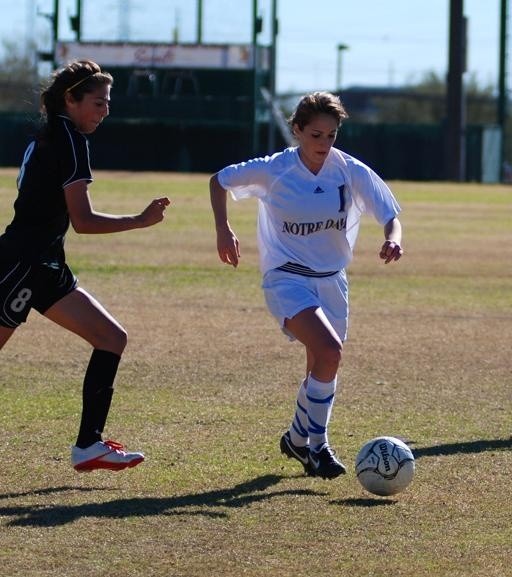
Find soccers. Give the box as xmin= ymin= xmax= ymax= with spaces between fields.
xmin=357 ymin=437 xmax=414 ymax=495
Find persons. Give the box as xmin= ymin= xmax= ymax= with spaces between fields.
xmin=0 ymin=60 xmax=170 ymax=472
xmin=208 ymin=91 xmax=403 ymax=480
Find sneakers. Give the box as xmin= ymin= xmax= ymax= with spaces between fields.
xmin=309 ymin=442 xmax=345 ymax=481
xmin=71 ymin=442 xmax=144 ymax=473
xmin=281 ymin=431 xmax=313 ymax=475
